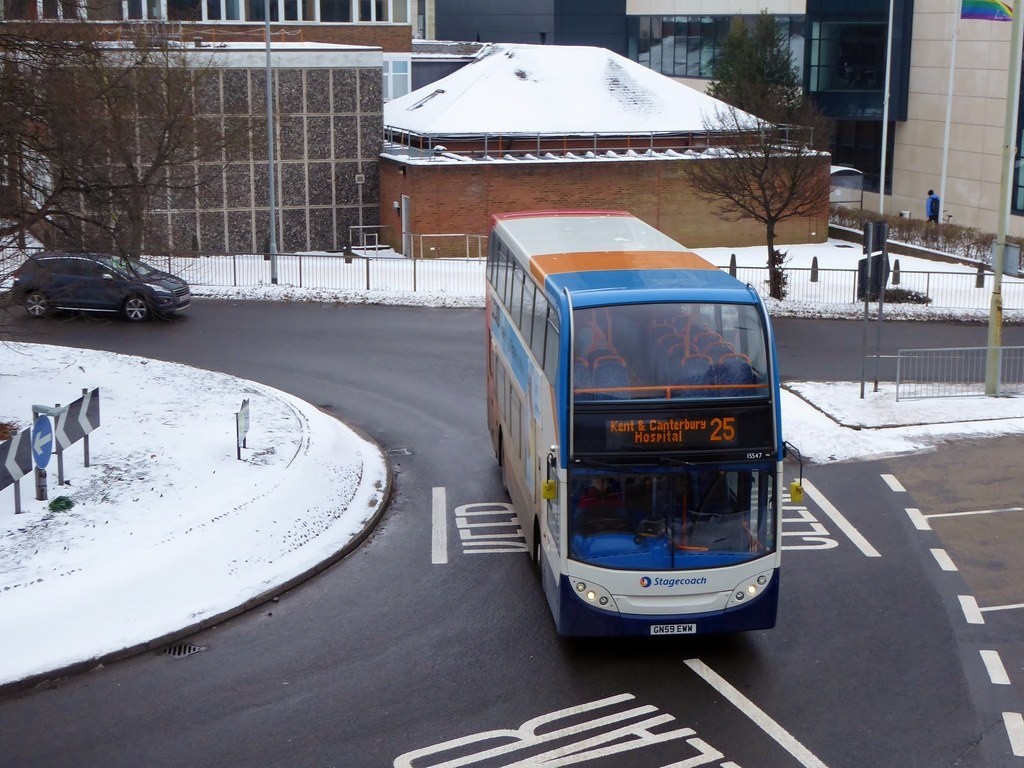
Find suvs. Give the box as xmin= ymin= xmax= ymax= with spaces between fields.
xmin=13 ymin=250 xmax=192 ymax=324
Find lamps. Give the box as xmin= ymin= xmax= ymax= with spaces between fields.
xmin=398 ymin=165 xmax=406 ymax=176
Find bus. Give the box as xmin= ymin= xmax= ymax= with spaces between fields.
xmin=482 ymin=207 xmax=807 ymax=640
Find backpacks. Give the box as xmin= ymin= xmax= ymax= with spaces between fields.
xmin=929 ymin=197 xmax=939 ymax=214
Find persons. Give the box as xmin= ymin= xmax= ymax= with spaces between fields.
xmin=575 ymin=475 xmax=628 ymax=533
xmin=921 ymin=189 xmax=940 ymax=242
xmin=579 ymin=313 xmax=607 ymax=359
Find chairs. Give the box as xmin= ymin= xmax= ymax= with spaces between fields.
xmin=572 ymin=311 xmax=754 ymax=401
